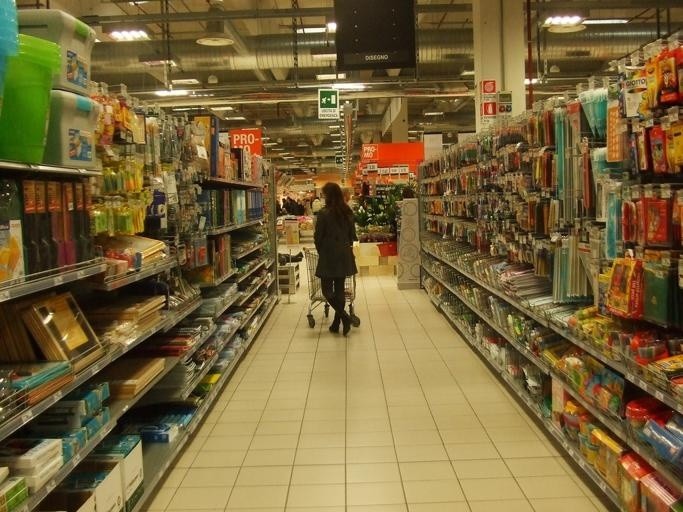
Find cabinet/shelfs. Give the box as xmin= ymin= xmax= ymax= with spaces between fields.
xmin=0 ymin=140 xmax=299 ymax=512
xmin=417 ymin=242 xmax=682 ymax=511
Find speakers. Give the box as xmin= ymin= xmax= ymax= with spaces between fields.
xmin=333 ymin=0 xmax=417 ymax=71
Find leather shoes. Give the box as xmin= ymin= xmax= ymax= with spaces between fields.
xmin=329 ymin=320 xmax=340 ymax=333
xmin=339 ymin=311 xmax=353 ymax=335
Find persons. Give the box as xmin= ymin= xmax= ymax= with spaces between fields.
xmin=275 ymin=195 xmax=321 ymax=219
xmin=312 ymin=182 xmax=359 ymax=336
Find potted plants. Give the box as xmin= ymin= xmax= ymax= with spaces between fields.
xmin=351 ymin=179 xmax=417 ymax=257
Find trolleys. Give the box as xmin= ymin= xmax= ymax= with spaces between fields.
xmin=301 ymin=246 xmax=361 ymax=328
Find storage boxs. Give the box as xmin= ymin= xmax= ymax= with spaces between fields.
xmin=19 ymin=7 xmax=102 ymax=170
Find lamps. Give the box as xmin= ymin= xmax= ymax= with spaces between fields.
xmin=193 ymin=6 xmax=236 ymax=48
xmin=548 ymin=64 xmax=561 ymax=73
xmin=539 ymin=7 xmax=634 ymax=29
xmin=100 ymin=22 xmax=157 ymax=43
xmin=292 ymin=19 xmax=351 ymax=82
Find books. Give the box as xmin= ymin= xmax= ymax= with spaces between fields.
xmin=195 ymin=187 xmax=264 ymax=229
